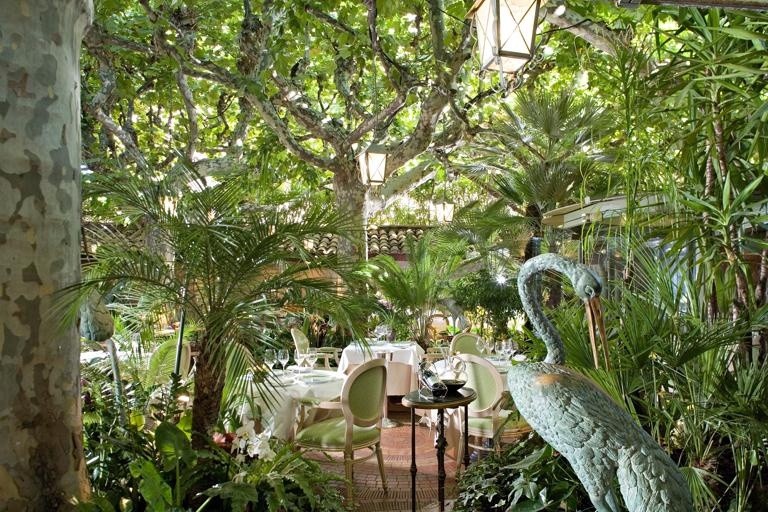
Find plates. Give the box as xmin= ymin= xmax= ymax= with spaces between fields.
xmin=266 ymin=377 xmax=297 ymax=386
xmin=300 ymin=373 xmax=338 ymax=383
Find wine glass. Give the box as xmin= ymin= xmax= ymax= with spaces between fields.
xmin=476 ymin=336 xmax=518 ymax=367
xmin=264 ymin=348 xmax=320 ymax=378
xmin=373 ymin=324 xmax=393 ymax=346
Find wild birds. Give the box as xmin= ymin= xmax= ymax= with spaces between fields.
xmin=505 ymin=252 xmax=697 ymax=511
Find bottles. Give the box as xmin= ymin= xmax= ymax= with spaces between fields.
xmin=418 ymin=362 xmax=447 ymax=400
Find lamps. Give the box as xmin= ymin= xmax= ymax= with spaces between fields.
xmin=355 ymin=53 xmax=388 ymax=186
xmin=435 ymin=110 xmax=456 ymax=224
xmin=464 ymin=0 xmax=548 ymax=95
